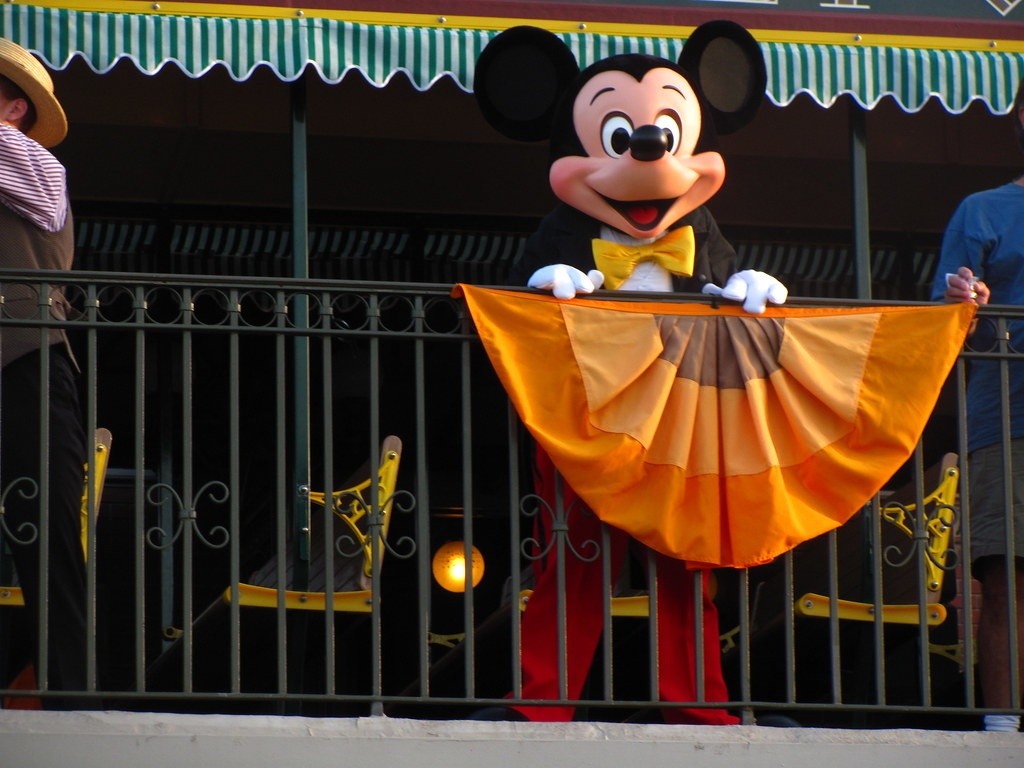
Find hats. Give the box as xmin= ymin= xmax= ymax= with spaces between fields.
xmin=0 ymin=37 xmax=68 ymax=148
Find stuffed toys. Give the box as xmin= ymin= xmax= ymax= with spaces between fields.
xmin=473 ymin=20 xmax=789 ymax=725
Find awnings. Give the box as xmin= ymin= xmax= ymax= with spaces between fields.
xmin=0 ymin=0 xmax=1024 ymax=117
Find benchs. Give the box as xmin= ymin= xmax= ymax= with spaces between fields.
xmin=0 ymin=428 xmax=113 ymax=605
xmin=405 ymin=568 xmax=651 ymax=699
xmin=704 ymin=452 xmax=958 ymax=700
xmin=108 ymin=435 xmax=401 ymax=689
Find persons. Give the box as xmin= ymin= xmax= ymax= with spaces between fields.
xmin=930 ymin=73 xmax=1024 ymax=732
xmin=0 ymin=37 xmax=91 ymax=710
